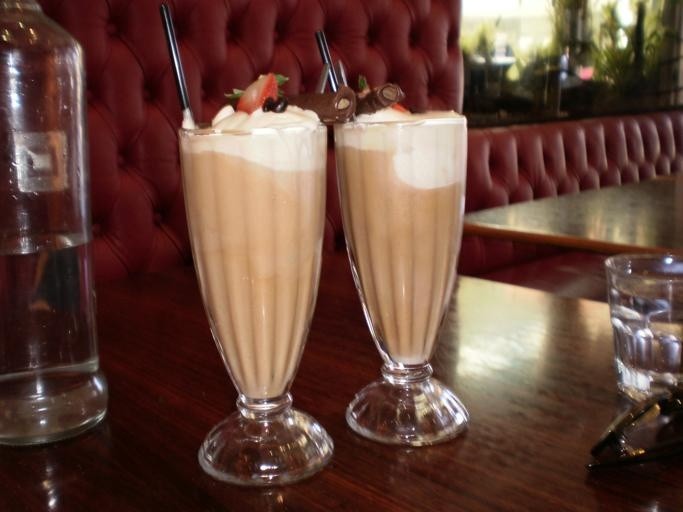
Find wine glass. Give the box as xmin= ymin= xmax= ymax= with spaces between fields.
xmin=175 ymin=111 xmax=331 ymax=485
xmin=336 ymin=113 xmax=471 ymax=446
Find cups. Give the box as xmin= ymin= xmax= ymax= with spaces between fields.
xmin=606 ymin=253 xmax=679 ymax=401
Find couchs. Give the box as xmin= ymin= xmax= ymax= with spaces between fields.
xmin=454 ymin=113 xmax=683 ymax=304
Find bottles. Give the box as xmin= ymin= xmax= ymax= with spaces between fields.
xmin=0 ymin=1 xmax=109 ymax=447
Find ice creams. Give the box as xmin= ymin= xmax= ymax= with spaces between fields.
xmin=333 ymin=83 xmax=468 ymax=366
xmin=177 ymin=70 xmax=357 ymax=399
